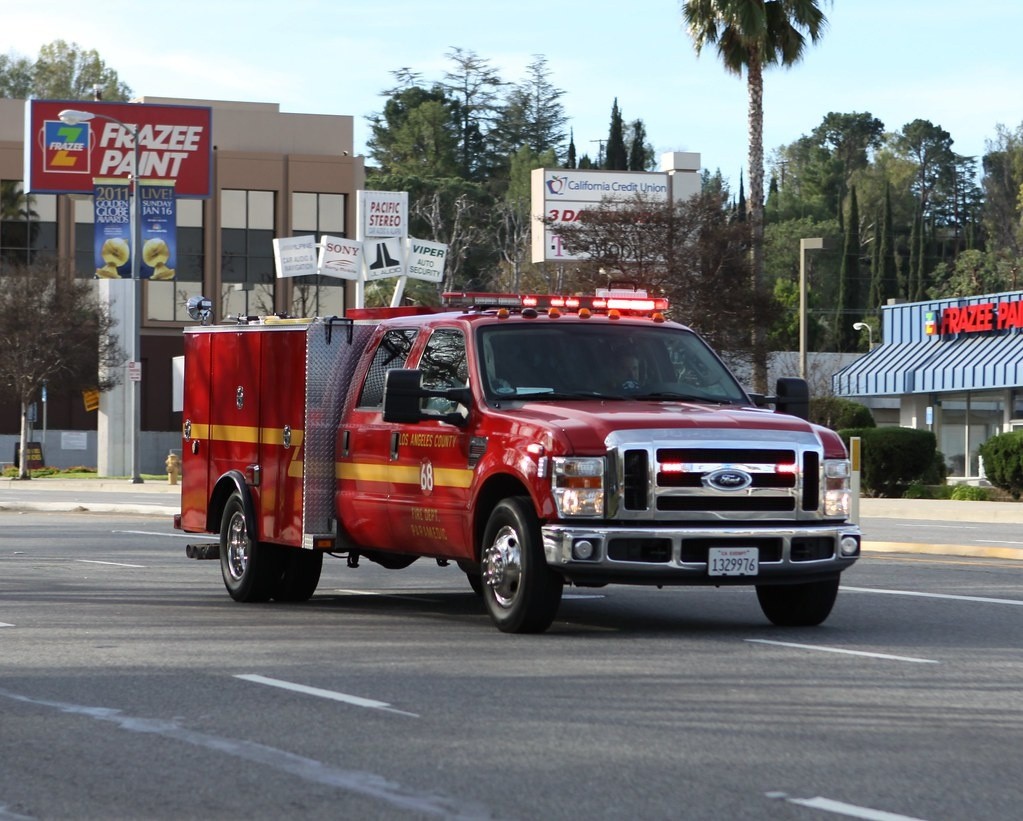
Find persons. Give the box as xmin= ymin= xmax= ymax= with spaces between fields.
xmin=608 ymin=351 xmax=640 ymax=391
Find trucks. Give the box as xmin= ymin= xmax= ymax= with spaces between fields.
xmin=171 ymin=287 xmax=866 ymax=635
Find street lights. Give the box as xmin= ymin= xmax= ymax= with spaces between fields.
xmin=56 ymin=107 xmax=143 ymax=484
xmin=165 ymin=454 xmax=181 ymax=486
xmin=852 ymin=322 xmax=873 ymax=352
